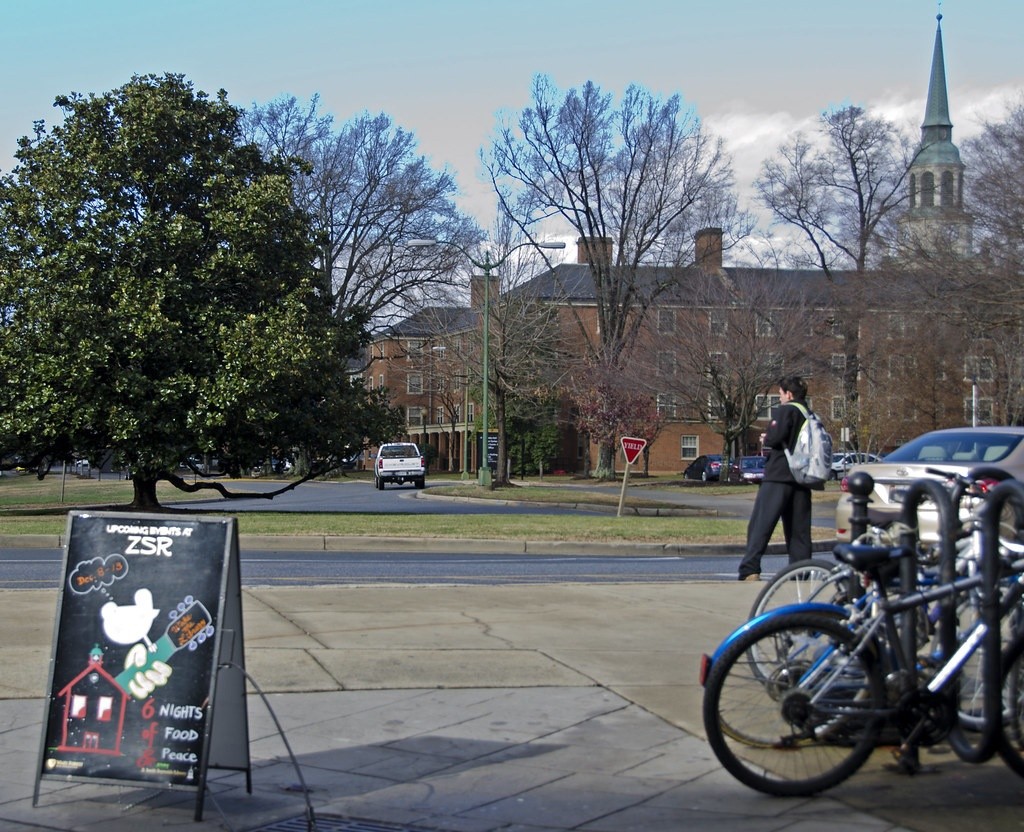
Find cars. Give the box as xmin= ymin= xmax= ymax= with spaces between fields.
xmin=837 ymin=425 xmax=1024 ymax=559
xmin=684 ymin=453 xmax=732 ymax=482
xmin=727 ymin=456 xmax=769 ymax=485
xmin=830 ymin=451 xmax=882 ymax=481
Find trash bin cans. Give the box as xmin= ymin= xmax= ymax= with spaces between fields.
xmin=76 ymin=460 xmax=90 ymax=478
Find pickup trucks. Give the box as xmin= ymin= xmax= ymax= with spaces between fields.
xmin=374 ymin=442 xmax=426 ymax=491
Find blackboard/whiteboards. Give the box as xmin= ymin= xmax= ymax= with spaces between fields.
xmin=41 ymin=511 xmax=252 ymax=789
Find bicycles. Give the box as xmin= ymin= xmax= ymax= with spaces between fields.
xmin=698 ymin=463 xmax=1024 ymax=798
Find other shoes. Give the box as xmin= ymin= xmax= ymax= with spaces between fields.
xmin=746 ymin=573 xmax=761 ymax=581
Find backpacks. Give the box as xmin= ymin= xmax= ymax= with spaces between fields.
xmin=780 ymin=402 xmax=833 ymax=484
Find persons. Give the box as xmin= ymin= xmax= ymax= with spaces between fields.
xmin=735 ymin=374 xmax=826 ymax=580
